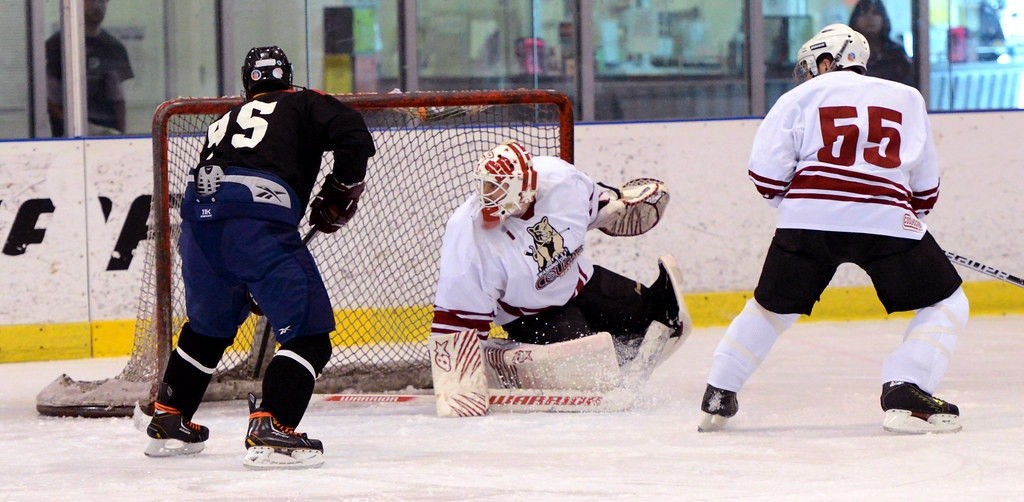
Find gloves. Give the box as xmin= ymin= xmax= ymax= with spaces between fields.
xmin=309 ymin=174 xmax=365 ymax=234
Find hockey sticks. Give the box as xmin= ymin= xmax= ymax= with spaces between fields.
xmin=130 ymin=220 xmax=324 ymax=433
xmin=309 ymin=318 xmax=676 ymax=414
xmin=942 ymin=249 xmax=1024 ymax=289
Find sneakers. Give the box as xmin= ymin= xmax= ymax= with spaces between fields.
xmin=143 ymin=379 xmax=209 ymax=457
xmin=880 ymin=381 xmax=962 ymax=434
xmin=243 ymin=391 xmax=325 ymax=470
xmin=697 ymin=383 xmax=739 ymax=434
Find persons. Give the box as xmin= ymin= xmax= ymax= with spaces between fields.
xmin=429 ymin=141 xmax=691 ymax=417
xmin=145 ymin=46 xmax=377 ymax=470
xmin=45 ymin=1 xmax=134 ymax=137
xmin=850 ymin=0 xmax=917 ymax=85
xmin=696 ymin=23 xmax=970 ymax=433
xmin=977 ymin=0 xmax=1004 ymax=61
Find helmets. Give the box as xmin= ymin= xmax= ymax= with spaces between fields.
xmin=470 ymin=138 xmax=537 ymax=232
xmin=241 ymin=45 xmax=293 ymax=100
xmin=791 ymin=24 xmax=870 ymax=87
